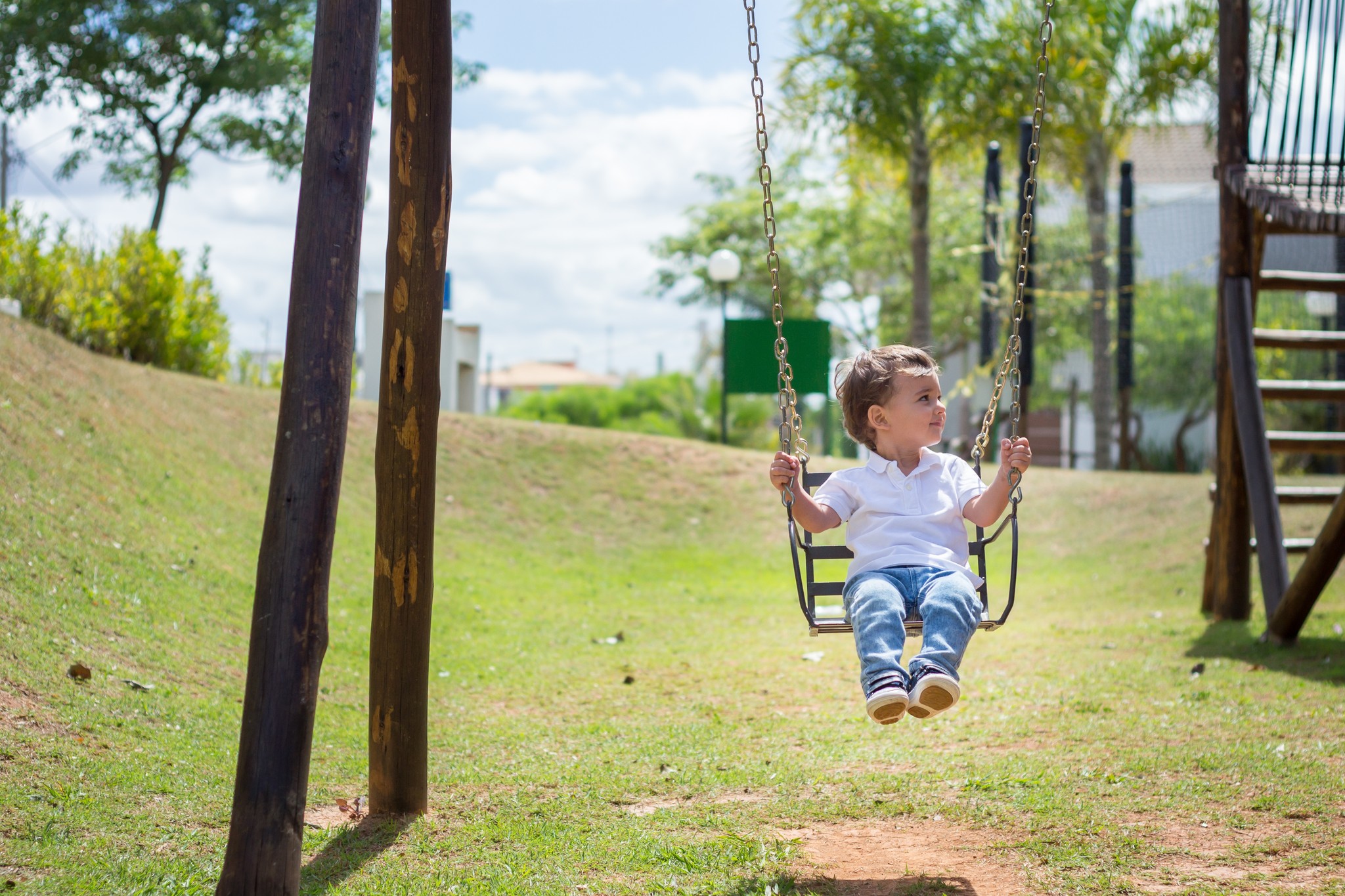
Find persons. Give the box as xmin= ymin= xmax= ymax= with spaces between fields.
xmin=769 ymin=343 xmax=1031 ymax=724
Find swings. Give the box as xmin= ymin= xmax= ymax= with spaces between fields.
xmin=743 ymin=0 xmax=1056 ymax=636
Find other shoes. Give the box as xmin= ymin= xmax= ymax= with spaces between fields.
xmin=866 ymin=683 xmax=910 ymax=725
xmin=906 ymin=672 xmax=961 ymax=718
